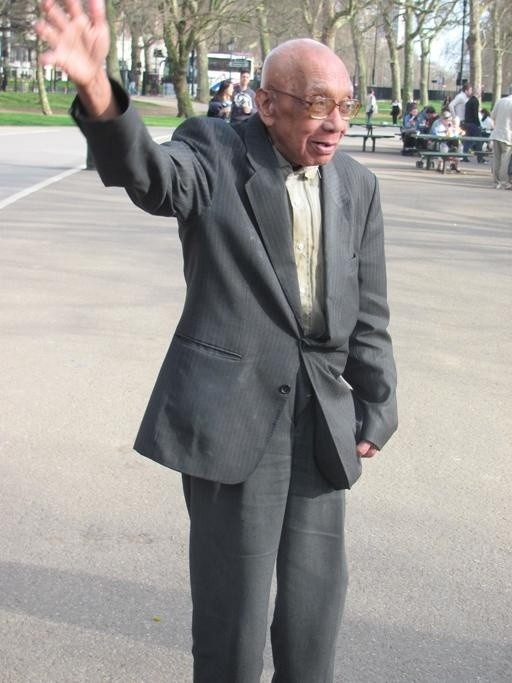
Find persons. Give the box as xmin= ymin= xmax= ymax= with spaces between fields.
xmin=365 ymin=88 xmax=376 ymax=123
xmin=33 ymin=1 xmax=399 ymax=683
xmin=205 ymin=64 xmax=261 ymax=125
xmin=401 ymin=83 xmax=512 ymax=190
xmin=390 ymin=98 xmax=402 ymax=126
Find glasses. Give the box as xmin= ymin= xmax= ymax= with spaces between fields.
xmin=266 ymin=88 xmax=361 ymax=119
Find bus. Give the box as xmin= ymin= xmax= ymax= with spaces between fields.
xmin=160 ymin=52 xmax=254 ymax=93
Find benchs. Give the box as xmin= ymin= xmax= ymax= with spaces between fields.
xmin=344 ymin=123 xmax=494 ymax=175
